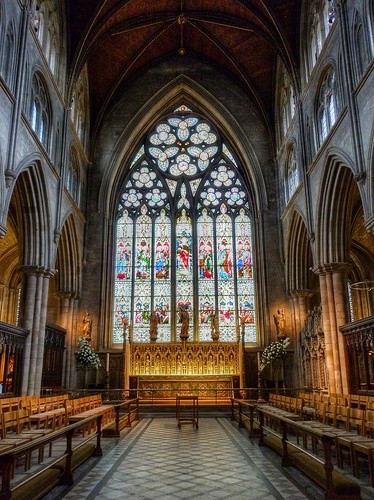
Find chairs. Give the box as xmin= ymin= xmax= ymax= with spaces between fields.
xmin=0 ymin=393 xmax=117 ymax=481
xmin=252 ymin=389 xmax=374 ymax=487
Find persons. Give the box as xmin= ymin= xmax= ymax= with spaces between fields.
xmin=179 ymin=305 xmax=189 ymax=335
xmin=212 ymin=317 xmax=219 ymax=333
xmin=147 ymin=311 xmax=158 ymax=339
xmin=116 ymin=235 xmax=255 ymax=325
xmin=0 ymin=381 xmax=4 ymax=394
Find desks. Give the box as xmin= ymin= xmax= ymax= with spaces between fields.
xmin=175 ymin=392 xmax=200 ymax=430
